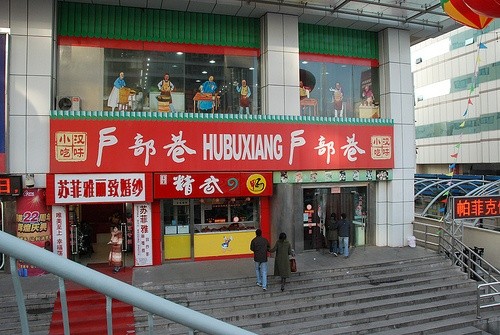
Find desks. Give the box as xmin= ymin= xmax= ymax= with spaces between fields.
xmin=354 ymin=105 xmax=380 ymax=119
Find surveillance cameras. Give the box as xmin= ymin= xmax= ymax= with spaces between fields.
xmin=25 ymin=176 xmax=34 ymax=188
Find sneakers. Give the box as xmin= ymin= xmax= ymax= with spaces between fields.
xmin=256 ymin=282 xmax=262 ymax=287
xmin=262 ymin=287 xmax=267 ymax=291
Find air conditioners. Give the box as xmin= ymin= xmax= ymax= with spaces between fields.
xmin=55 ymin=95 xmax=82 ymax=111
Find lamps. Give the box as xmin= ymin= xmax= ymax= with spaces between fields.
xmin=24 ymin=173 xmax=35 ymax=187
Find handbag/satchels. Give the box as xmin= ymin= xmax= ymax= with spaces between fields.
xmin=289 ymin=259 xmax=297 ymax=272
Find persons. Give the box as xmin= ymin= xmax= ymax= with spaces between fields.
xmin=269 ymin=233 xmax=291 ymax=291
xmin=250 ymin=229 xmax=270 ymax=291
xmin=326 ymin=213 xmax=350 ymax=258
xmin=108 ymin=227 xmax=122 ymax=273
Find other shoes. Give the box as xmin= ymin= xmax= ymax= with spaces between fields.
xmin=281 ymin=285 xmax=285 ymax=291
xmin=329 ymin=251 xmax=349 ymax=259
xmin=113 ymin=266 xmax=120 ymax=273
